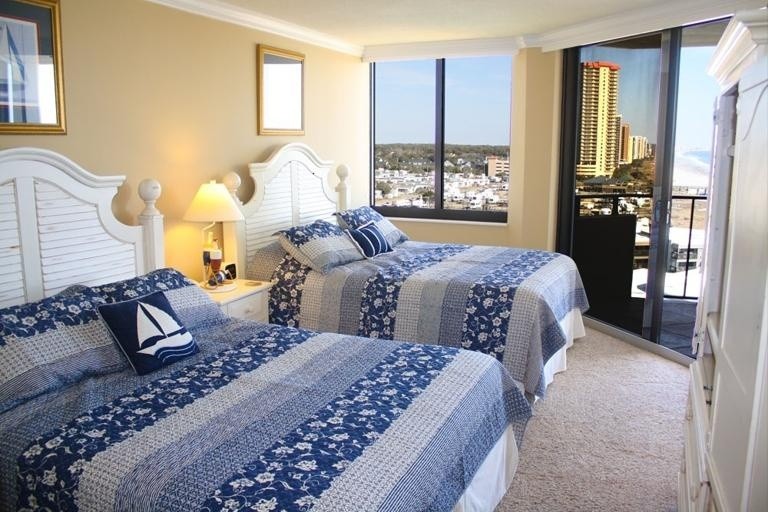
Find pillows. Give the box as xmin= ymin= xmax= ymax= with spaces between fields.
xmin=97 ymin=288 xmax=201 ymax=376
xmin=274 ymin=202 xmax=412 ymax=274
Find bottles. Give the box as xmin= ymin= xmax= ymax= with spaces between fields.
xmin=209 ymin=270 xmax=229 ymax=285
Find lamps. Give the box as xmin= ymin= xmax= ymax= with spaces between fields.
xmin=180 ymin=183 xmax=248 ymax=291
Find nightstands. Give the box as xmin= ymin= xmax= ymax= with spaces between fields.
xmin=195 ymin=276 xmax=272 ymax=324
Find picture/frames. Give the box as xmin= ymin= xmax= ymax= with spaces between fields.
xmin=255 ymin=42 xmax=309 ymax=138
xmin=0 ymin=0 xmax=66 ymax=135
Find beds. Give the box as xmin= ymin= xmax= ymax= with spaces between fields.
xmin=1 ymin=147 xmax=512 ymax=512
xmin=220 ymin=140 xmax=577 ymax=405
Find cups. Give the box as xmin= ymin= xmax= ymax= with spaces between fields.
xmin=210 ymin=250 xmax=221 ymax=273
